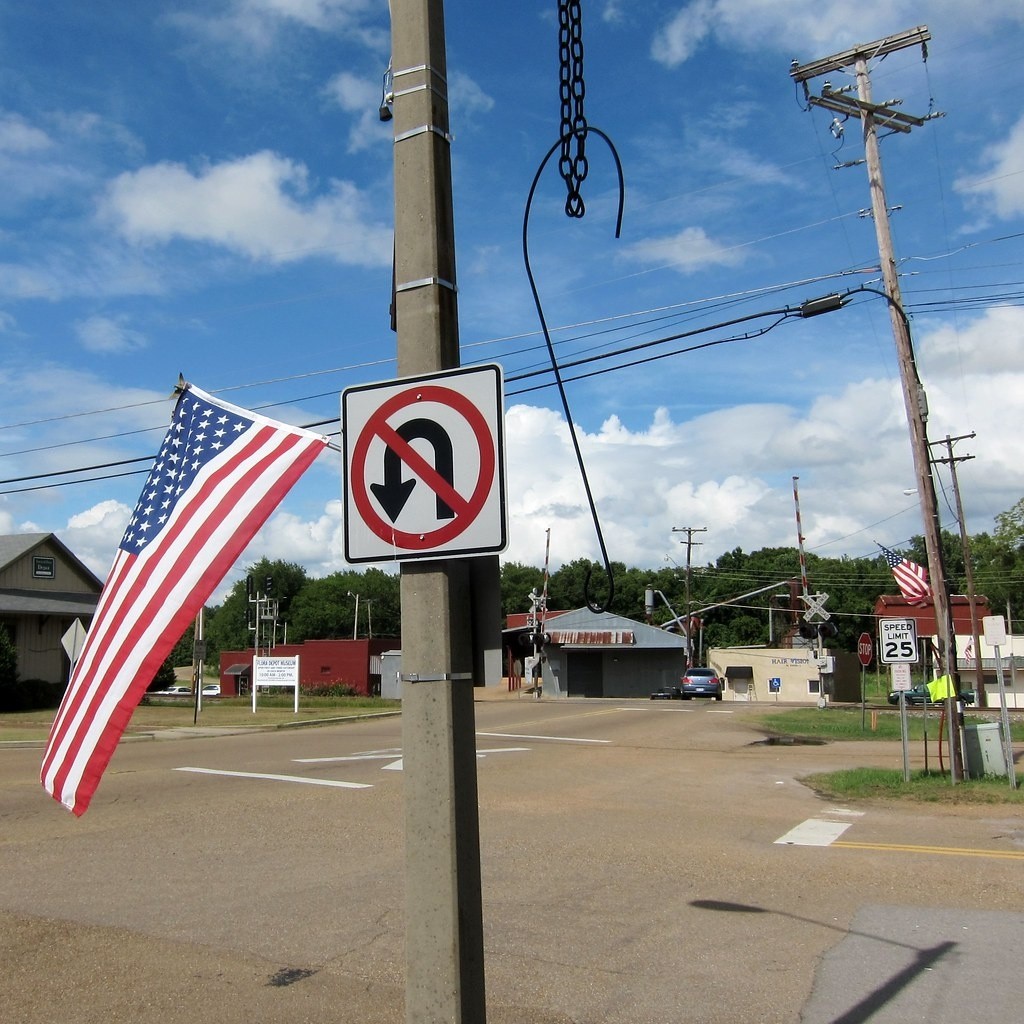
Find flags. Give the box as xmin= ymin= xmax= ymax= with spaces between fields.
xmin=41 ymin=385 xmax=328 ymax=818
xmin=964 ymin=635 xmax=975 ymax=668
xmin=876 ymin=539 xmax=933 ymax=606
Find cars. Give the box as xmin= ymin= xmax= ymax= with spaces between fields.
xmin=681 ymin=668 xmax=724 ymax=701
xmin=888 ymin=682 xmax=974 ymax=706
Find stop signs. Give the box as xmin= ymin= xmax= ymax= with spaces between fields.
xmin=857 ymin=632 xmax=872 ymax=666
